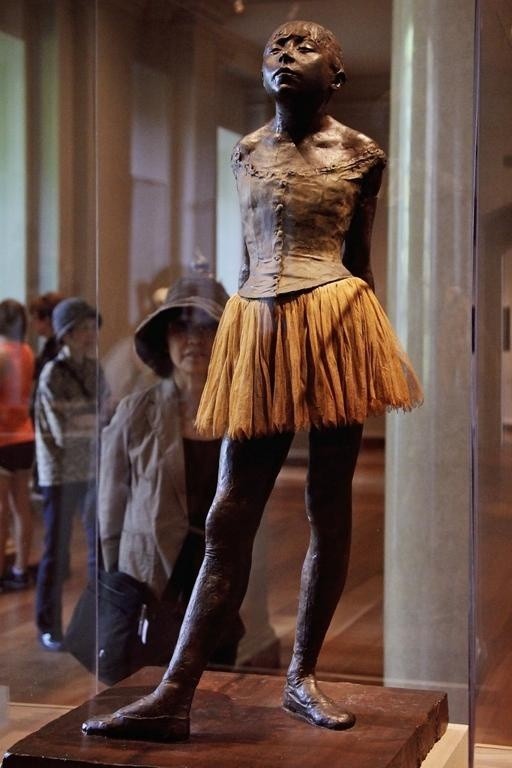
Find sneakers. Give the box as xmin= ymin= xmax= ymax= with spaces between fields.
xmin=37 ymin=627 xmax=66 ymax=652
xmin=10 ymin=569 xmax=29 ymax=591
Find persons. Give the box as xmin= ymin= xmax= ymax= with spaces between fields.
xmin=95 ymin=275 xmax=231 ymax=675
xmin=80 ymin=18 xmax=425 ymax=743
xmin=33 ymin=298 xmax=113 ymax=654
xmin=0 ymin=298 xmax=36 ymax=592
xmin=27 ymin=290 xmax=70 ymax=583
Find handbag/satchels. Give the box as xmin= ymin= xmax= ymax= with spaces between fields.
xmin=64 ymin=571 xmax=156 ymax=682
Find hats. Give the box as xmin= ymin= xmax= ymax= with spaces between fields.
xmin=49 ymin=299 xmax=103 ymax=342
xmin=133 ymin=277 xmax=234 ymax=377
